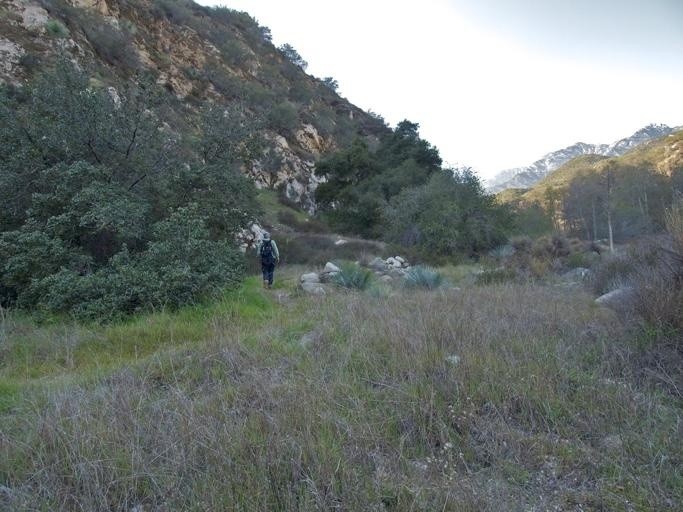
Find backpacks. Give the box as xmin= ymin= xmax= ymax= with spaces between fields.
xmin=261 ymin=240 xmax=272 ymax=257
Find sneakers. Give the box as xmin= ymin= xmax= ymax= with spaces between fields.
xmin=264 ymin=280 xmax=272 ymax=289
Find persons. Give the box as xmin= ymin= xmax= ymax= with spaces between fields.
xmin=258 ymin=232 xmax=280 ymax=288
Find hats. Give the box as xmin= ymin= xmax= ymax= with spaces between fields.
xmin=264 ymin=232 xmax=271 ymax=238
